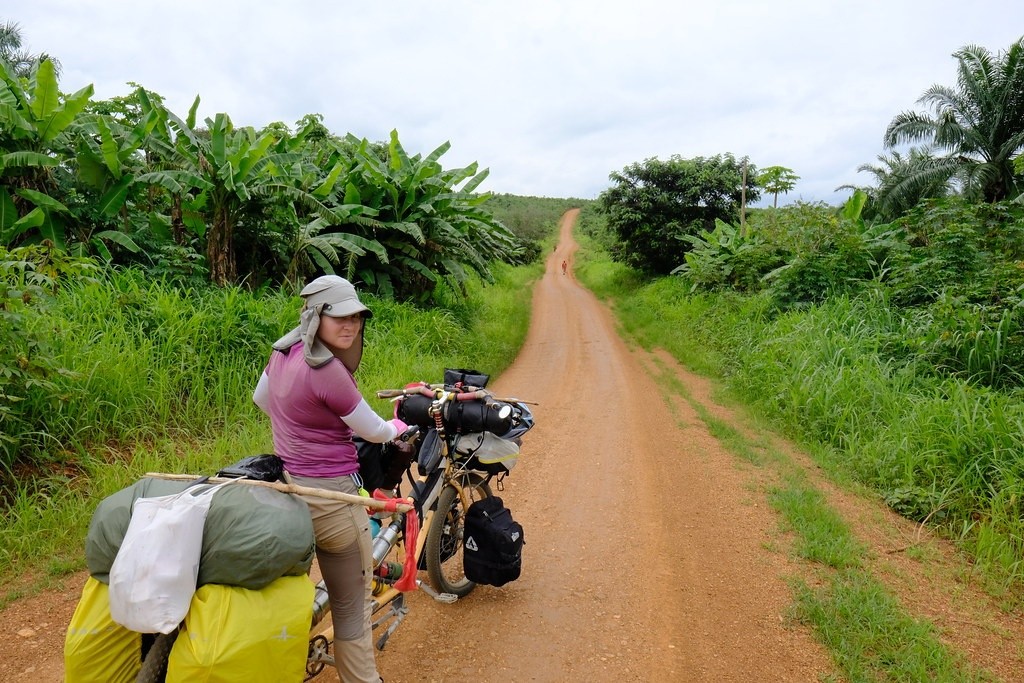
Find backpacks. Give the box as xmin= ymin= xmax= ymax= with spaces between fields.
xmin=462 ymin=495 xmax=526 ymax=587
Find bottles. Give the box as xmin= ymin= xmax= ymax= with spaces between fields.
xmin=375 ymin=562 xmax=402 ymax=580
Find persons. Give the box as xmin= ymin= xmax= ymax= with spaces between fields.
xmin=562 ymin=260 xmax=567 ymax=275
xmin=251 ymin=275 xmax=406 ymax=683
xmin=553 ymin=246 xmax=558 ymax=256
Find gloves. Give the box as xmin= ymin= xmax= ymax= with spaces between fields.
xmin=387 ymin=419 xmax=407 ymax=439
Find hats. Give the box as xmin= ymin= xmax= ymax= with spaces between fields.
xmin=272 ymin=275 xmax=373 ymax=375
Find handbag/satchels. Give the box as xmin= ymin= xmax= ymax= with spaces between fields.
xmin=109 ymin=476 xmax=248 ymax=634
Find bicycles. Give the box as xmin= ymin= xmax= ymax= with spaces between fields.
xmin=126 ymin=379 xmax=514 ymax=682
